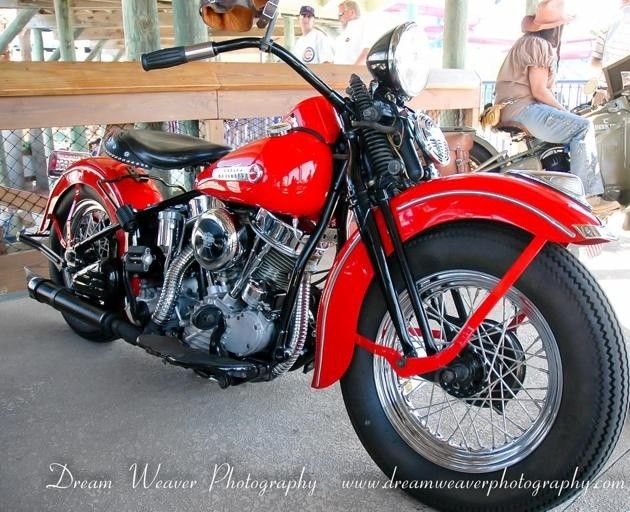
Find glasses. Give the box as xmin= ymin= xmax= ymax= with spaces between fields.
xmin=338 ymin=11 xmax=347 ymax=16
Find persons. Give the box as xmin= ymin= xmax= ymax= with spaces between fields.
xmin=493 ymin=1 xmax=620 ymax=215
xmin=290 ymin=5 xmax=332 ymax=64
xmin=332 ymin=0 xmax=376 ymax=67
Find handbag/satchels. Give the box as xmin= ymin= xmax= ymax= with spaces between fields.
xmin=479 ymin=103 xmax=502 ymax=128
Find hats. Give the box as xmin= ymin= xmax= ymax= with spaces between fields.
xmin=300 ymin=6 xmax=315 ymax=17
xmin=520 ymin=0 xmax=577 ymax=32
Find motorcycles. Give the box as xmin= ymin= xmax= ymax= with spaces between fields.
xmin=16 ymin=1 xmax=630 ymax=510
xmin=442 ymin=56 xmax=630 ymax=223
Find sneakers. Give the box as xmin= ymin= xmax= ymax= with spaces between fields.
xmin=587 ymin=196 xmax=620 ymax=214
